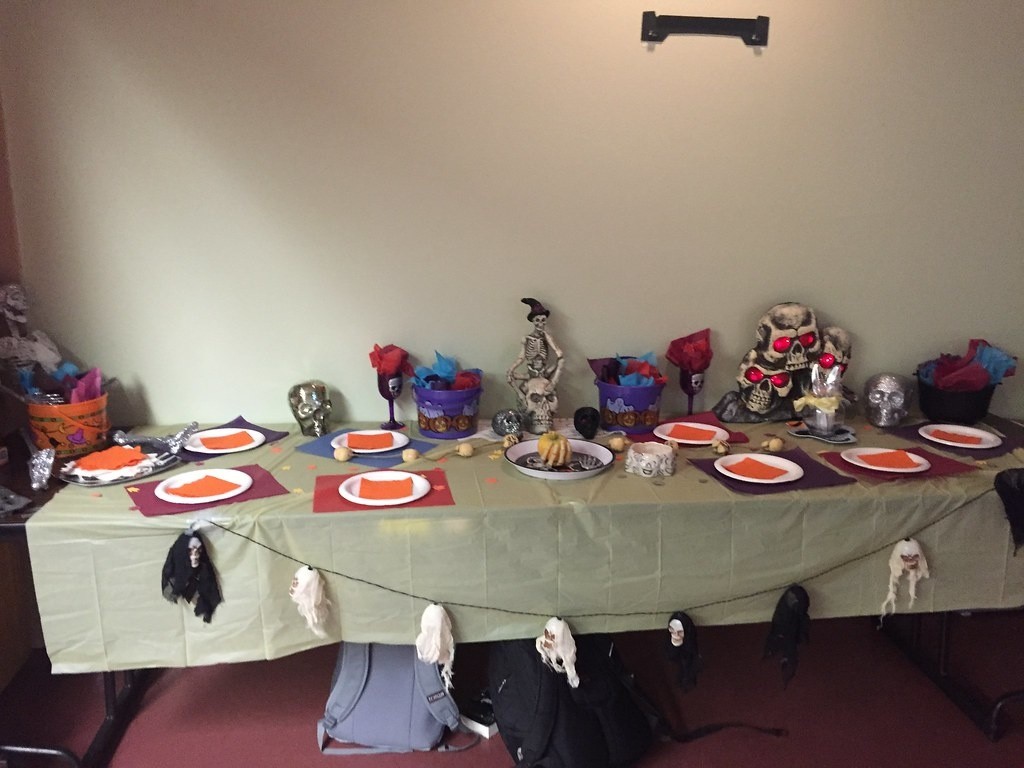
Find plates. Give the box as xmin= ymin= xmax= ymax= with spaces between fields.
xmin=155 ymin=469 xmax=253 ymax=504
xmin=841 ymin=447 xmax=932 ymax=473
xmin=182 ymin=428 xmax=266 ymax=453
xmin=339 ymin=470 xmax=430 ymax=505
xmin=714 ymin=453 xmax=804 ymax=483
xmin=331 ymin=430 xmax=409 ymax=454
xmin=504 ymin=439 xmax=614 ymax=480
xmin=918 ymin=424 xmax=1003 ymax=449
xmin=653 ymin=422 xmax=729 ymax=445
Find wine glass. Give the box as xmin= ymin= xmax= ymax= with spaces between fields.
xmin=680 ymin=367 xmax=704 ymax=416
xmin=378 ymin=371 xmax=404 ymax=430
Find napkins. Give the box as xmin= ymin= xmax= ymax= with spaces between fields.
xmin=858 ymin=449 xmax=921 ymax=468
xmin=348 ymin=433 xmax=393 ymax=449
xmin=200 ymin=431 xmax=253 ymax=450
xmin=668 ymin=424 xmax=716 ymax=441
xmin=721 ymin=457 xmax=788 ymax=479
xmin=358 ymin=477 xmax=413 ymax=500
xmin=59 ymin=442 xmax=157 ymax=482
xmin=930 ymin=427 xmax=981 ymax=444
xmin=167 ymin=474 xmax=239 ymax=497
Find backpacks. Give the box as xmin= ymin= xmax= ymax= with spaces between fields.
xmin=488 ymin=633 xmax=788 ymax=768
xmin=316 ymin=642 xmax=480 ymax=754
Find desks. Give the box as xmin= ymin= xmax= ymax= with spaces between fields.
xmin=0 ymin=412 xmax=1024 ymax=768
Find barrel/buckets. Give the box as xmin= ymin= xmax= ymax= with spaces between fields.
xmin=25 ymin=392 xmax=111 ymax=459
xmin=596 ymin=381 xmax=666 ymax=433
xmin=410 ymin=382 xmax=483 ymax=439
